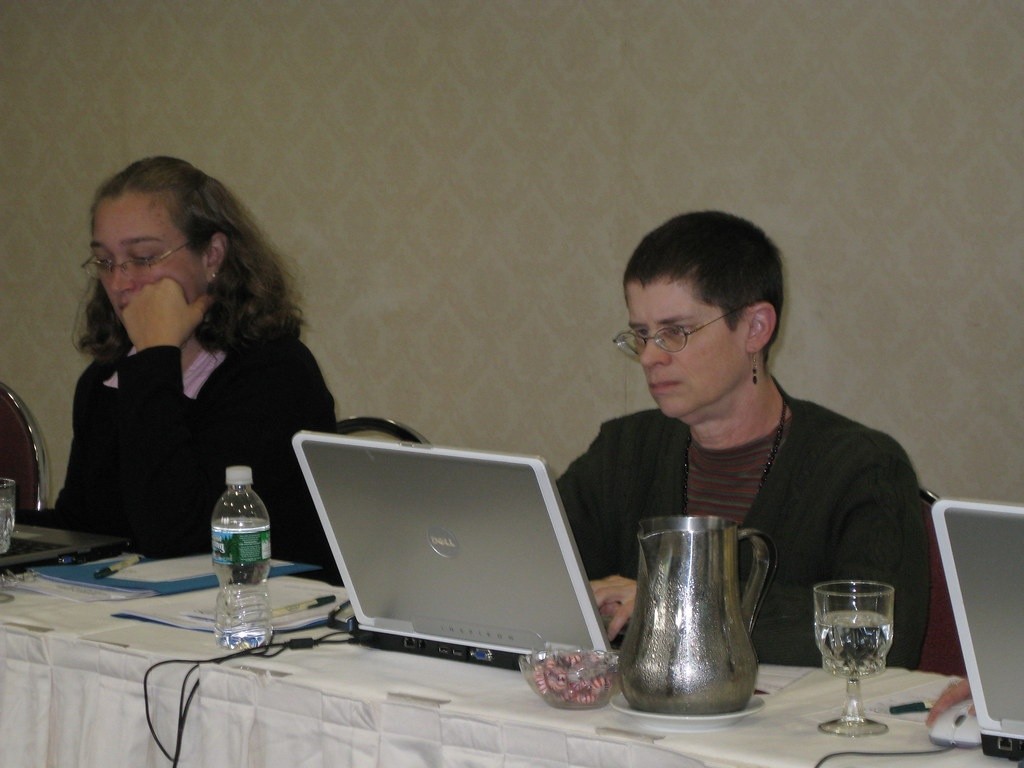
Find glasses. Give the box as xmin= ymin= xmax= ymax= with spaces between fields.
xmin=81 ymin=237 xmax=195 ymax=280
xmin=613 ymin=303 xmax=751 ymax=360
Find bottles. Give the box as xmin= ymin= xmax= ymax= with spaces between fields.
xmin=210 ymin=465 xmax=273 ymax=651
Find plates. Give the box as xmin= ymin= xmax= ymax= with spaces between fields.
xmin=609 ymin=690 xmax=766 ymax=732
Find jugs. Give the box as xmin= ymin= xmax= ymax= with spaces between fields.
xmin=614 ymin=514 xmax=779 ymax=716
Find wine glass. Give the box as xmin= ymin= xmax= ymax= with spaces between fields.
xmin=811 ymin=579 xmax=902 ymax=734
xmin=0 ymin=478 xmax=16 ymax=604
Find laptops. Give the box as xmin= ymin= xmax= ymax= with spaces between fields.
xmin=292 ymin=430 xmax=628 ymax=668
xmin=931 ymin=497 xmax=1024 ymax=762
xmin=0 ymin=521 xmax=130 ymax=575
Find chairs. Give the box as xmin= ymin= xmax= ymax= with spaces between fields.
xmin=918 ymin=489 xmax=965 ymax=678
xmin=0 ymin=382 xmax=48 ymax=513
xmin=337 ymin=415 xmax=430 ymax=445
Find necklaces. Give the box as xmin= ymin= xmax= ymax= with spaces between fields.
xmin=681 ymin=404 xmax=786 ymax=514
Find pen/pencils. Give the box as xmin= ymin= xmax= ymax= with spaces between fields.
xmin=890 ymin=699 xmax=938 ymax=715
xmin=94 ymin=554 xmax=142 ymax=579
xmin=273 ymin=595 xmax=336 ymax=616
xmin=328 ymin=600 xmax=350 ymax=616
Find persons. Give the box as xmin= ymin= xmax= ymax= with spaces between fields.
xmin=14 ymin=156 xmax=338 ymax=568
xmin=926 ymin=677 xmax=977 ymax=727
xmin=555 ymin=212 xmax=933 ymax=670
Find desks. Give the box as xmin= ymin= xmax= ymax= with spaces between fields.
xmin=0 ymin=559 xmax=1024 ymax=768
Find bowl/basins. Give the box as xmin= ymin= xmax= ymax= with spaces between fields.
xmin=518 ymin=648 xmax=620 ymax=709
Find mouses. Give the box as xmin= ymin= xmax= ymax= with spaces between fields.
xmin=328 ymin=599 xmax=357 ymax=633
xmin=927 ymin=699 xmax=983 ymax=749
